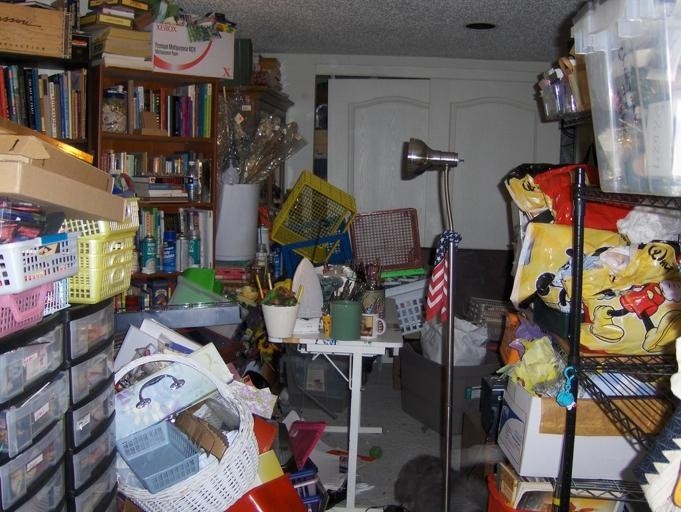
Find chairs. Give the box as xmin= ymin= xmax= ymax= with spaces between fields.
xmin=402 ymin=246 xmax=514 ymax=435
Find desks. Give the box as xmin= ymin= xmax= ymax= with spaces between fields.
xmin=268 ymin=296 xmax=404 ymax=512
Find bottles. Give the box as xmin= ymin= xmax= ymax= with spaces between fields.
xmin=141 ymin=227 xmax=279 ymax=286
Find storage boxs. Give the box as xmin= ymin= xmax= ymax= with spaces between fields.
xmin=494 ymin=376 xmax=679 ymax=481
xmin=144 ymin=22 xmax=235 ymax=80
xmin=570 ymin=0 xmax=681 ymax=198
xmin=488 ymin=472 xmax=593 ymax=512
xmin=398 ymin=344 xmax=503 ymax=438
xmin=0 ymin=1 xmax=74 ymax=60
xmin=0 ymin=296 xmax=116 ymax=511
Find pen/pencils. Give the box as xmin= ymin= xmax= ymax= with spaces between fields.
xmin=327 ymin=278 xmax=370 ymax=301
xmin=353 ymin=255 xmax=384 ymax=289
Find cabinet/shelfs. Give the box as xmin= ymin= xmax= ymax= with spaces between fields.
xmin=215 ymin=85 xmax=293 ymax=213
xmin=0 ymin=52 xmax=96 ymax=167
xmin=253 ymin=52 xmax=562 ymax=251
xmin=89 ymin=61 xmax=216 ymax=281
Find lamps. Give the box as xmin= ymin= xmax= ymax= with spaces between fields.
xmin=405 ymin=137 xmax=464 ymax=512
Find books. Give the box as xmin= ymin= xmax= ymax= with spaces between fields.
xmin=140 ymin=206 xmax=213 ymax=271
xmin=103 ymin=147 xmax=210 ymax=204
xmin=0 ymin=1 xmax=215 ymax=137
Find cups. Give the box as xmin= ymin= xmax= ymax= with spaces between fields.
xmin=261 ymin=303 xmax=299 ymax=338
xmin=330 ymin=299 xmax=362 ymax=342
xmin=361 ymin=312 xmax=387 ymax=340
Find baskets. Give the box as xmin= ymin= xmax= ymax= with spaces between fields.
xmin=0 ymin=197 xmax=141 ymax=337
xmin=271 ymin=170 xmax=357 ymax=280
xmin=286 ymin=457 xmax=321 ymax=512
xmin=384 ymin=278 xmax=428 ymax=336
xmin=117 ymin=354 xmax=259 ymax=512
xmin=350 ymin=207 xmax=423 ymax=271
xmin=467 ymin=297 xmax=514 ymax=323
